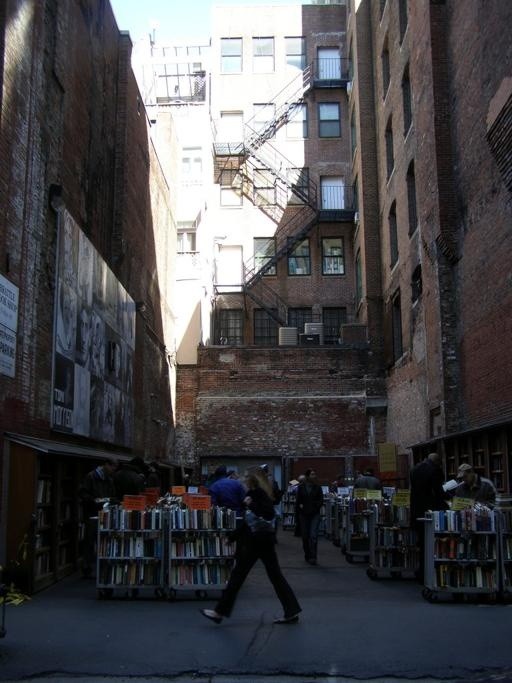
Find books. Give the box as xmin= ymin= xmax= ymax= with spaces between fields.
xmin=433 ymin=509 xmax=512 ymax=588
xmin=99 ymin=488 xmax=237 ymax=585
xmin=282 ymin=486 xmax=298 ymax=525
xmin=442 ymin=479 xmax=465 ymax=493
xmin=323 ymin=497 xmax=411 ymax=569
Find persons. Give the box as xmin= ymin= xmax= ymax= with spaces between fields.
xmin=81 ymin=457 xmax=125 ymax=579
xmin=204 ymin=465 xmax=276 ymax=566
xmin=147 ymin=463 xmax=167 ymax=497
xmin=411 ymin=453 xmax=456 ymax=580
xmin=199 ymin=466 xmax=303 ymax=623
xmin=76 ymin=301 xmax=92 ymax=368
xmin=455 ymin=463 xmax=497 ymax=509
xmin=354 ymin=465 xmax=380 ymax=489
xmin=336 ymin=475 xmax=345 ymax=487
xmin=295 ymin=468 xmax=322 ymax=564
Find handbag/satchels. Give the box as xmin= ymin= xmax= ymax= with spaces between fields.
xmin=239 ymin=508 xmax=277 ymax=544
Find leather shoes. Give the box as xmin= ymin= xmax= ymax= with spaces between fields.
xmin=272 ymin=615 xmax=300 ymax=625
xmin=197 ymin=607 xmax=223 ymax=625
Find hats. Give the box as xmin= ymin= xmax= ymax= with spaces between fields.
xmin=212 ymin=465 xmax=227 ymax=477
xmin=127 ymin=457 xmax=145 ymax=466
xmin=457 ymin=463 xmax=473 ymax=477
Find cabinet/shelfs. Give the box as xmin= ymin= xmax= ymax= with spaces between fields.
xmin=319 ymin=497 xmax=333 ymax=536
xmin=499 ymin=508 xmax=512 ymax=603
xmin=93 ymin=507 xmax=165 ymax=599
xmin=280 ymin=491 xmax=305 ymax=531
xmin=367 ymin=499 xmax=423 ymax=583
xmin=343 ymin=497 xmax=382 ymax=566
xmin=420 ymin=505 xmax=499 ymax=607
xmin=165 ymin=506 xmax=238 ymax=602
